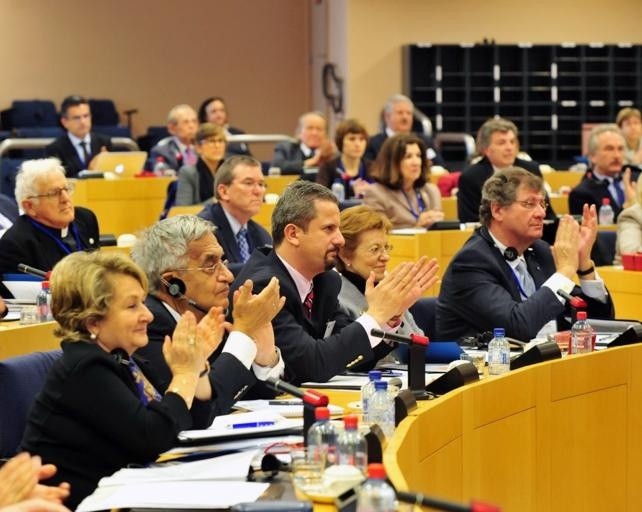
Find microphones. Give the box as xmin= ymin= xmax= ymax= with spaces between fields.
xmin=557 ymin=291 xmax=575 ymax=302
xmin=336 ymin=167 xmax=345 ymax=178
xmin=372 ymin=328 xmax=415 ymax=344
xmin=265 ymin=378 xmax=304 ymax=398
xmin=18 ymin=263 xmax=47 ymax=278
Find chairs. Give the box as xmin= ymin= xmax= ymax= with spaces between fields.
xmin=300 ymin=173 xmax=317 ymax=182
xmin=409 ymin=298 xmax=435 ymax=341
xmin=0 ymin=158 xmax=22 ymax=196
xmin=0 ymin=194 xmax=20 ymax=223
xmin=87 ymin=99 xmax=119 ymax=127
xmin=0 ymin=351 xmax=63 ymax=456
xmin=148 ymin=127 xmax=168 ymax=152
xmin=592 ymin=231 xmax=617 ymax=265
xmin=16 ymin=128 xmax=66 ymax=159
xmin=91 ymin=126 xmax=131 ymax=151
xmin=159 ymin=181 xmax=177 ymax=220
xmin=12 ymin=101 xmax=58 ymax=128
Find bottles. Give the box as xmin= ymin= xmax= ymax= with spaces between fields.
xmin=570 ymin=313 xmax=591 ymax=353
xmin=307 ymin=407 xmax=336 ymax=467
xmin=356 ymin=464 xmax=399 ymax=512
xmin=175 ymin=153 xmax=187 ymax=175
xmin=341 ymin=176 xmax=355 ymax=201
xmin=335 ymin=416 xmax=368 ymax=465
xmin=368 ymin=382 xmax=394 ymax=437
xmin=330 ymin=176 xmax=344 ymax=201
xmin=599 ymin=198 xmax=613 ymax=226
xmin=364 ymin=371 xmax=381 ymax=419
xmin=36 ymin=283 xmax=54 ymax=321
xmin=487 ymin=328 xmax=510 ymax=375
xmin=153 ymin=156 xmax=166 ymax=176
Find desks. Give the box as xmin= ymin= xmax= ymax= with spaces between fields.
xmin=1 ymin=321 xmax=60 ymax=359
xmin=598 ymin=267 xmax=642 ymax=320
xmin=70 ymin=172 xmax=584 ymax=240
xmin=112 ymin=330 xmax=642 ymax=512
xmin=169 ymin=198 xmax=568 ymax=233
xmin=385 ymin=226 xmax=619 ymax=297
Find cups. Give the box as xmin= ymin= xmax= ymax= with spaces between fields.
xmin=290 ymin=444 xmax=329 ymax=501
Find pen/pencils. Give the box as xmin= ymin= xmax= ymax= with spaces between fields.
xmin=269 ymin=401 xmax=303 ymax=405
xmin=233 ymin=421 xmax=275 ymax=429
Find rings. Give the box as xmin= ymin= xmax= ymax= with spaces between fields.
xmin=272 ymin=303 xmax=277 ymax=310
xmin=187 ymin=339 xmax=194 ymax=345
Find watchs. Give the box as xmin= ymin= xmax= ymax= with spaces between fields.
xmin=576 ymin=260 xmax=596 ymax=276
xmin=199 ymin=360 xmax=210 ymax=379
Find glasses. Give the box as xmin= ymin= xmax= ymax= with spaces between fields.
xmin=27 ymin=181 xmax=76 ymax=201
xmin=352 ymin=242 xmax=394 ymax=255
xmin=516 ymin=198 xmax=548 ymax=212
xmin=171 ymin=259 xmax=229 ymax=273
xmin=230 ymin=179 xmax=268 ymax=192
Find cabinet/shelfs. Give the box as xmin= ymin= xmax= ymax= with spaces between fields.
xmin=401 ymin=44 xmax=642 ymax=170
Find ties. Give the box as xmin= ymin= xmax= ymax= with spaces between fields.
xmin=80 ymin=142 xmax=91 ymax=166
xmin=303 ymin=288 xmax=313 ymax=321
xmin=237 ymin=225 xmax=252 ymax=263
xmin=186 ymin=147 xmax=197 ymax=167
xmin=517 ymin=260 xmax=536 ymax=301
xmin=613 ymin=178 xmax=627 ymax=207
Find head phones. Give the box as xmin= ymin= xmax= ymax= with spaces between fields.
xmin=157 ymin=278 xmax=230 ymax=321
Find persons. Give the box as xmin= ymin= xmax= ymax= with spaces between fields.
xmin=0 ymin=157 xmax=100 ymax=275
xmin=175 ymin=124 xmax=231 ymax=207
xmin=457 ymin=119 xmax=560 ymax=222
xmin=364 ymin=132 xmax=446 ymax=228
xmin=45 ymin=94 xmax=116 ymax=174
xmin=131 ymin=214 xmax=289 ymax=416
xmin=618 ymin=173 xmax=642 ymax=254
xmin=315 ymin=119 xmax=373 ymax=200
xmin=435 ymin=167 xmax=615 ymax=341
xmin=198 ymin=98 xmax=246 ymax=135
xmin=19 ymin=251 xmax=226 ymax=512
xmin=152 ymin=103 xmax=200 ymax=177
xmin=196 ymin=156 xmax=274 ymax=263
xmin=570 ymin=125 xmax=637 ymax=266
xmin=334 ymin=204 xmax=428 ymax=365
xmin=272 ymin=112 xmax=341 ymax=176
xmin=1 ymin=444 xmax=75 ymax=512
xmin=617 ymin=109 xmax=641 ymax=167
xmin=225 ymin=181 xmax=441 ymax=386
xmin=362 ymin=94 xmax=444 ymax=167
